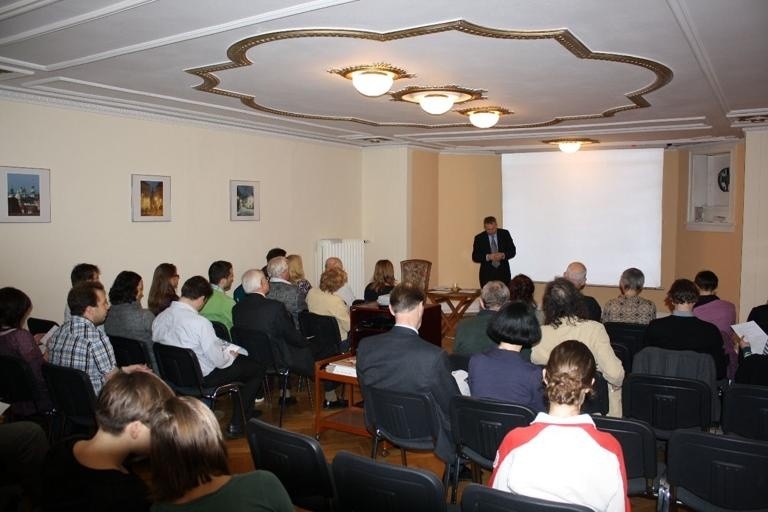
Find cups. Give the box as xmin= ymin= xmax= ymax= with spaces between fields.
xmin=451 ymin=283 xmax=460 ymax=293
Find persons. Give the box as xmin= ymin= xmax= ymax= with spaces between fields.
xmin=364 ymin=260 xmax=395 ymax=304
xmin=0 ymin=249 xmax=351 ymax=511
xmin=647 ymin=270 xmax=768 ymax=385
xmin=356 ymin=283 xmax=480 ymax=489
xmin=471 ymin=216 xmax=517 ymax=290
xmin=455 ymin=262 xmax=656 ymax=512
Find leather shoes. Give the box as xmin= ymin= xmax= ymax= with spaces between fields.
xmin=324 ymin=399 xmax=349 ymax=408
xmin=279 ymin=396 xmax=296 ymax=405
xmin=442 ymin=465 xmax=484 ymax=481
xmin=226 ymin=419 xmax=243 ymax=438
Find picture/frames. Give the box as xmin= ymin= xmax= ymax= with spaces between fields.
xmin=228 ymin=180 xmax=262 ymax=222
xmin=131 ymin=173 xmax=171 ymax=222
xmin=0 ymin=165 xmax=52 ymax=224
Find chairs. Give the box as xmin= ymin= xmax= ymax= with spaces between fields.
xmin=1 ymin=259 xmax=768 ymax=512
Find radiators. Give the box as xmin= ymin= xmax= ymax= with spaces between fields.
xmin=314 ymin=237 xmax=366 ymax=300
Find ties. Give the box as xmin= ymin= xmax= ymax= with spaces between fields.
xmin=491 ymin=235 xmax=500 ymax=268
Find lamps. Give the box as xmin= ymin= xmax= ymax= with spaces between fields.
xmin=328 ymin=63 xmax=417 ymax=98
xmin=544 ymin=137 xmax=599 ymax=153
xmin=456 ymin=105 xmax=517 ymax=130
xmin=388 ymin=82 xmax=488 ymax=116
